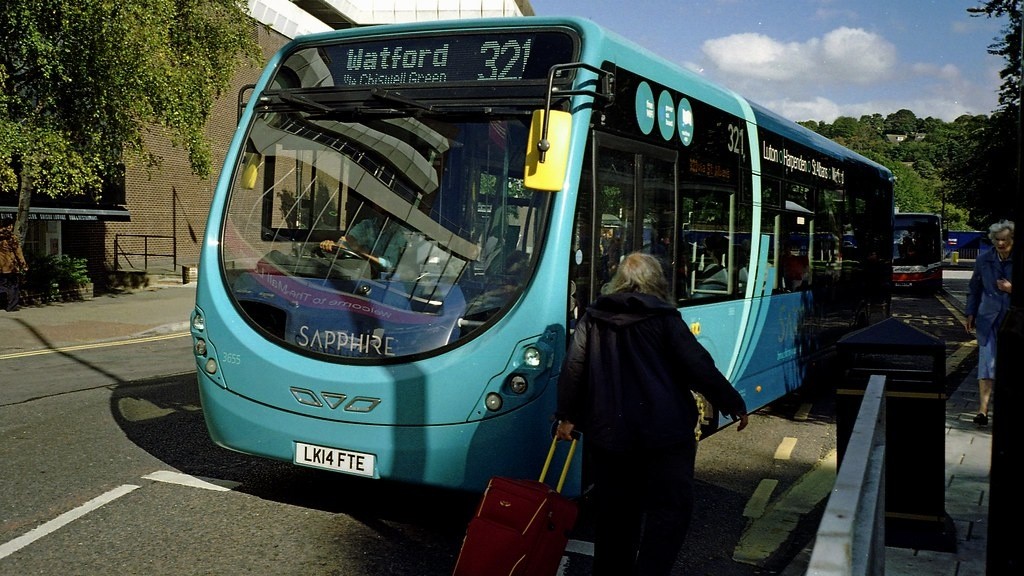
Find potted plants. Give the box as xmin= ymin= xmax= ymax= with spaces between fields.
xmin=68 ymin=256 xmax=95 ymax=302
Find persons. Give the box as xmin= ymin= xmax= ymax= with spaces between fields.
xmin=965 ymin=221 xmax=1014 ymax=428
xmin=555 ymin=252 xmax=749 ymax=576
xmin=0 ymin=217 xmax=30 ymax=311
xmin=320 ymin=198 xmax=411 ymax=281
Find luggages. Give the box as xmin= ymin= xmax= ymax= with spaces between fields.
xmin=453 ymin=428 xmax=581 ymax=576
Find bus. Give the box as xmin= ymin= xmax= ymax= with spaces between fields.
xmin=890 ymin=212 xmax=950 ymax=293
xmin=602 ymin=226 xmax=834 ymax=292
xmin=186 ymin=15 xmax=898 ymax=496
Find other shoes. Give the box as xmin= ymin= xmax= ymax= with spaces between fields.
xmin=973 ymin=413 xmax=989 ymax=426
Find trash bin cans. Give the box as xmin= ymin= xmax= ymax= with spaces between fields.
xmin=837 ymin=318 xmax=958 ymax=553
